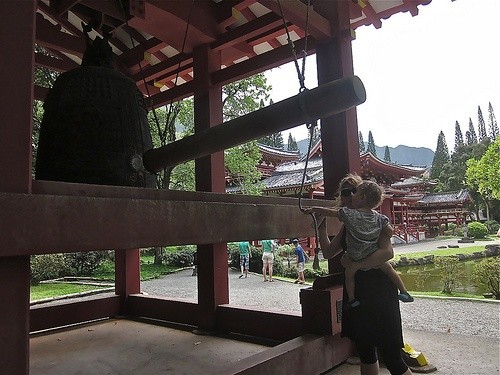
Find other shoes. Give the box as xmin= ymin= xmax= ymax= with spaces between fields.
xmin=264 ymin=279 xmax=268 ymax=282
xmin=298 ymin=281 xmax=306 ymax=284
xmin=295 ymin=279 xmax=301 ymax=283
xmin=347 ymin=299 xmax=361 ymax=307
xmin=269 ymin=280 xmax=274 ymax=282
xmin=245 ymin=276 xmax=249 ymax=278
xmin=398 ymin=293 xmax=414 ymax=302
xmin=239 ymin=275 xmax=243 ymax=278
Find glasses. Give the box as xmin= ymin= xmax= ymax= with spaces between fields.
xmin=341 ymin=188 xmax=358 ymax=197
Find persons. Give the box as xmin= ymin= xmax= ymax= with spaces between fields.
xmin=261 ymin=240 xmax=278 ymax=282
xmin=314 ymin=171 xmax=413 ymax=375
xmin=239 ymin=241 xmax=253 ymax=278
xmin=303 ymin=180 xmax=414 ymax=309
xmin=290 ymin=239 xmax=309 ymax=284
